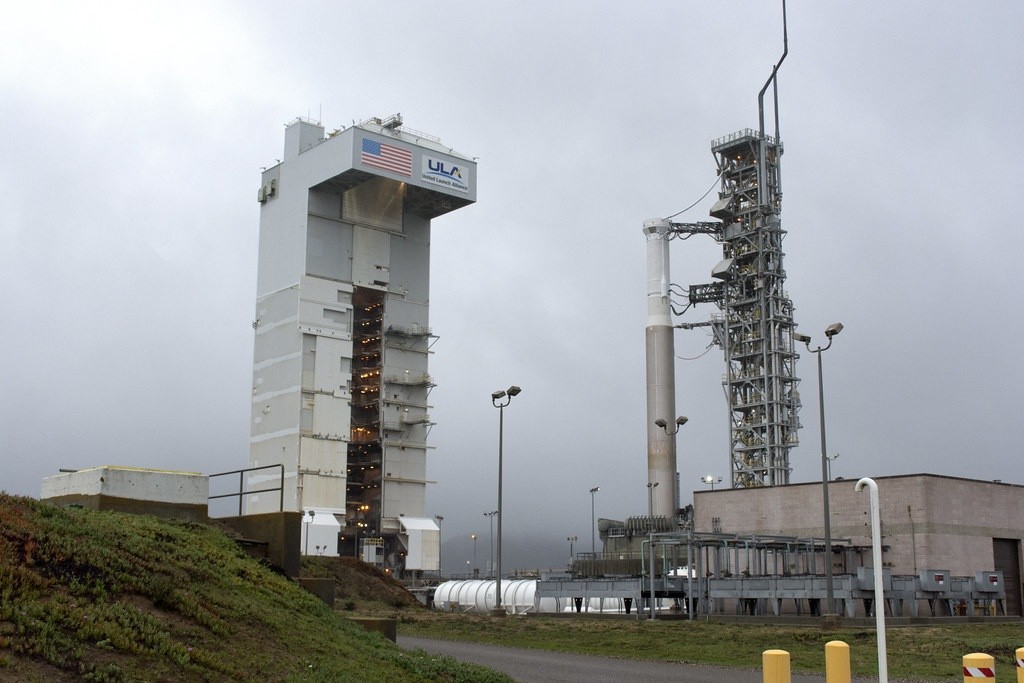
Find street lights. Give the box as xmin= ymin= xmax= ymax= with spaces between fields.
xmin=646 ymin=481 xmax=659 ymax=517
xmin=791 ymin=321 xmax=845 ymax=629
xmin=490 ymin=385 xmax=522 ymax=617
xmin=471 ymin=533 xmax=477 ymax=569
xmin=434 ymin=514 xmax=444 ymax=582
xmin=590 ymin=487 xmax=600 ymax=553
xmin=655 ymin=416 xmax=689 ymax=613
xmin=826 ymin=454 xmax=841 ymax=482
xmin=567 ymin=536 xmax=578 ymax=558
